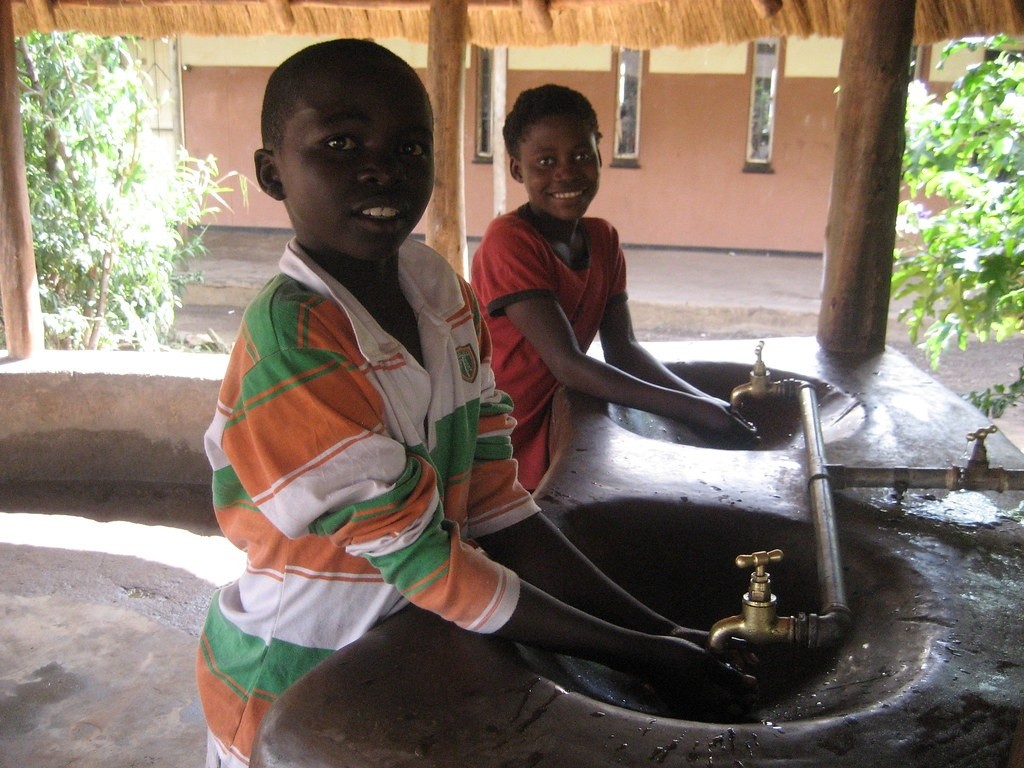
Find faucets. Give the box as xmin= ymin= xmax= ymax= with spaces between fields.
xmin=728 ymin=340 xmax=785 ymax=411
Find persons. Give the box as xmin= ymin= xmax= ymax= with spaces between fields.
xmin=196 ymin=39 xmax=756 ymax=768
xmin=470 ymin=84 xmax=761 ymax=494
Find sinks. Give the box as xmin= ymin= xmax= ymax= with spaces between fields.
xmin=521 ymin=500 xmax=939 ymax=726
xmin=708 ymin=549 xmax=799 ymax=652
xmin=601 ymin=358 xmax=867 ymax=449
